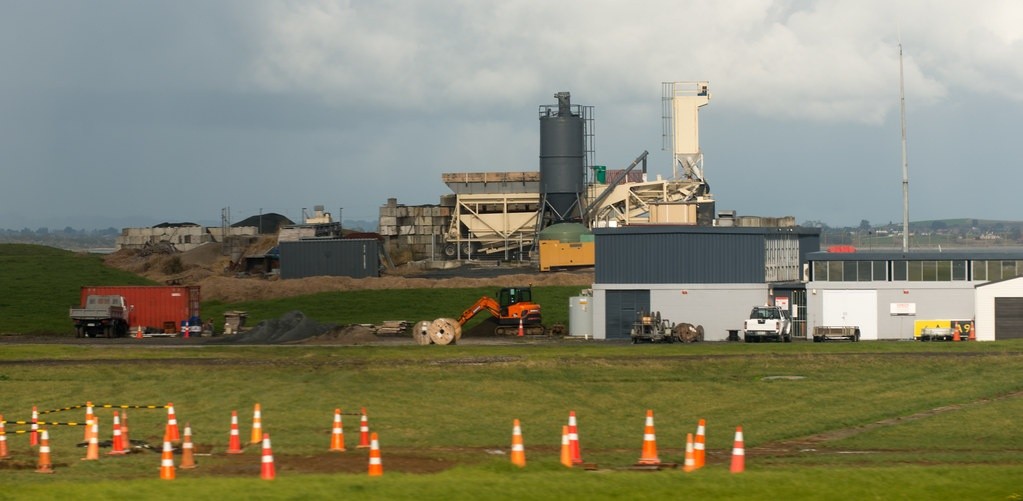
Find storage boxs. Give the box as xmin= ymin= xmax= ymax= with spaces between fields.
xmin=80 ymin=285 xmax=201 ymax=335
xmin=280 ymin=238 xmax=381 ymax=279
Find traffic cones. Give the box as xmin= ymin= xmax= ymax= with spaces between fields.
xmin=356 ymin=406 xmax=372 ymax=448
xmin=260 ymin=433 xmax=276 ymax=480
xmin=560 ymin=409 xmax=583 ymax=467
xmin=136 ymin=325 xmax=143 ymax=339
xmin=683 ymin=418 xmax=707 ymax=472
xmin=0 ymin=401 xmax=180 ymax=472
xmin=729 ymin=426 xmax=746 ymax=473
xmin=250 ymin=403 xmax=264 ymax=444
xmin=366 ymin=432 xmax=383 ymax=476
xmin=176 ymin=421 xmax=200 ymax=469
xmin=226 ymin=410 xmax=243 ymax=455
xmin=328 ymin=408 xmax=347 ymax=452
xmin=518 ymin=317 xmax=524 ymax=337
xmin=639 ymin=408 xmax=662 ymax=465
xmin=185 ymin=321 xmax=189 ymax=338
xmin=511 ymin=418 xmax=526 ymax=468
xmin=161 ymin=434 xmax=176 ymax=480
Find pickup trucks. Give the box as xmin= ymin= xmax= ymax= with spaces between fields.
xmin=744 ymin=305 xmax=792 ymax=343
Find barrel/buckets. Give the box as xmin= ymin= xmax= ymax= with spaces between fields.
xmin=163 ymin=321 xmax=176 ymax=333
xmin=591 ymin=165 xmax=605 ymax=185
xmin=208 ymin=323 xmax=215 ymax=333
xmin=181 ymin=317 xmax=204 ymax=337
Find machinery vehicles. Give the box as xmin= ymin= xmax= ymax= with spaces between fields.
xmin=458 ymin=281 xmax=543 ymax=337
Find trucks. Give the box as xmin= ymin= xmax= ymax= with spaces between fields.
xmin=69 ymin=293 xmax=134 ymax=337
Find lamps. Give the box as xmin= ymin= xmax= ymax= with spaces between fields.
xmin=812 ymin=289 xmax=816 ymax=294
